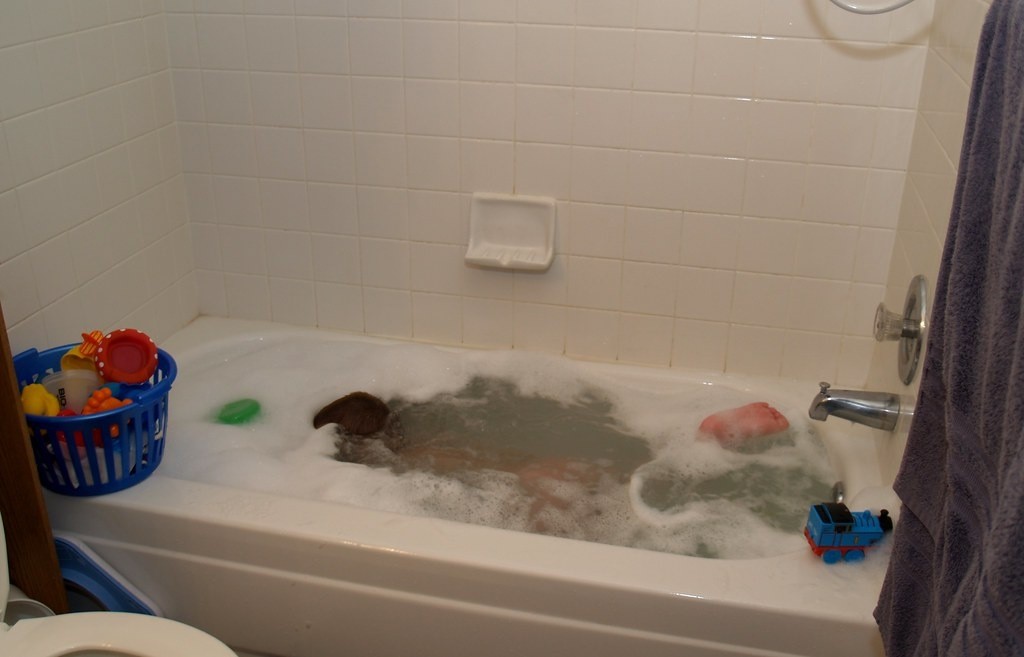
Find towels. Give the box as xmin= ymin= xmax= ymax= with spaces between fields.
xmin=871 ymin=0 xmax=1021 ymax=657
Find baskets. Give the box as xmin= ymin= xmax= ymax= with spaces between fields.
xmin=12 ymin=342 xmax=178 ymax=497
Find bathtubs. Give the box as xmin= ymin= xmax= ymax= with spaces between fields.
xmin=44 ymin=310 xmax=889 ymax=657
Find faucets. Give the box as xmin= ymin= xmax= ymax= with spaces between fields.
xmin=806 ymin=381 xmax=901 ymax=432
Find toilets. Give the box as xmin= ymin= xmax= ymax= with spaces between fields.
xmin=0 ymin=512 xmax=241 ymax=657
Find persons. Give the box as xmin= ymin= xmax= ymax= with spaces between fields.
xmin=313 ymin=391 xmax=789 ymax=440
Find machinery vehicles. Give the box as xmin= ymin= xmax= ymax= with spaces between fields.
xmin=805 ymin=503 xmax=893 ymax=564
